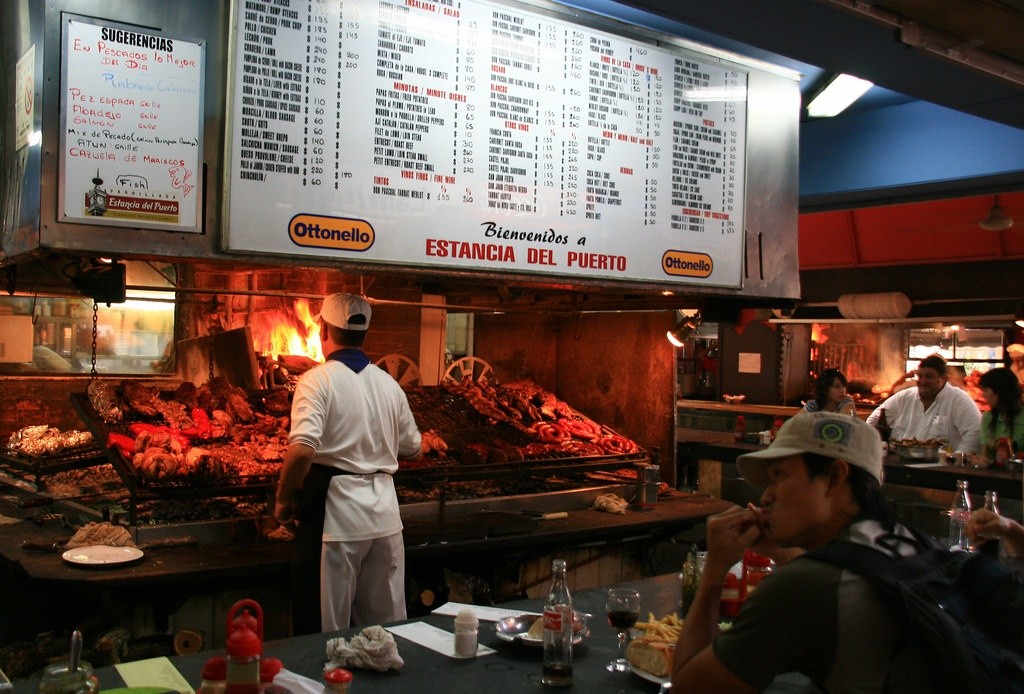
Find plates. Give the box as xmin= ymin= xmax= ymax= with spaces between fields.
xmin=629 ymin=661 xmax=672 ymax=685
xmin=495 ymin=613 xmax=582 ymax=647
xmin=61 ymin=545 xmax=144 ymax=565
xmin=744 ymin=432 xmax=760 ymax=443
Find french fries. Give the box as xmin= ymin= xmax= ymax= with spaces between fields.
xmin=632 ymin=611 xmax=685 ymax=643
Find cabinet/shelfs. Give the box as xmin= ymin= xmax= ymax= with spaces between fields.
xmin=677 ymin=399 xmax=871 ymax=506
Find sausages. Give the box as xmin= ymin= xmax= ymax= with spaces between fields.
xmin=527 ymin=418 xmax=637 ymax=454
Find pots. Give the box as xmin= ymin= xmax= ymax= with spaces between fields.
xmin=888 ymin=437 xmax=950 ymax=464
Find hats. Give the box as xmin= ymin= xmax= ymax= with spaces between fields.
xmin=737 ymin=413 xmax=884 ymax=487
xmin=313 ymin=292 xmax=372 ymax=331
xmin=1006 ymin=343 xmax=1024 ymax=358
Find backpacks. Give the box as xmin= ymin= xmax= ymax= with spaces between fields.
xmin=796 ymin=523 xmax=1024 ymax=694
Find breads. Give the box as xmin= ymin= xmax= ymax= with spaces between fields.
xmin=526 ymin=617 xmax=584 ymax=639
xmin=626 ymin=638 xmax=675 ymax=677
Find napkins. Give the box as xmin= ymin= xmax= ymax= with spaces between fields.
xmin=272 ymin=668 xmax=325 ymax=694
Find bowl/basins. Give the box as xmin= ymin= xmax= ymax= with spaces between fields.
xmin=725 ymin=398 xmax=741 ymax=404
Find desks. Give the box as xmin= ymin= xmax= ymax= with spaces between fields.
xmin=0 ymin=572 xmax=812 ymax=694
xmin=883 ymin=453 xmax=1024 ymax=501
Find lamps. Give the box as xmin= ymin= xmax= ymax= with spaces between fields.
xmin=978 ymin=194 xmax=1014 ymax=233
xmin=1015 ymin=317 xmax=1024 ymax=328
xmin=939 ymin=329 xmax=951 ymax=350
xmin=666 ymin=309 xmax=702 ymax=348
xmin=71 ymin=258 xmax=127 ymax=307
xmin=804 ymin=72 xmax=875 ymax=120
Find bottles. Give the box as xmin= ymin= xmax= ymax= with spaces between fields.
xmin=977 ymin=490 xmax=1002 ymax=563
xmin=676 ymin=551 xmax=708 ymax=623
xmin=454 ymin=609 xmax=479 ymax=658
xmin=848 ymin=408 xmax=856 ymax=418
xmin=734 ymin=416 xmax=782 ymax=445
xmin=322 ymin=669 xmax=354 ymax=694
xmin=938 ymin=437 xmax=1016 ymax=472
xmin=875 ymin=407 xmax=890 ymax=458
xmin=33 ymin=297 xmax=81 ymax=317
xmin=706 ymin=376 xmax=711 ymax=387
xmin=200 ymin=599 xmax=289 ymax=694
xmin=721 ymin=550 xmax=776 ymax=618
xmin=949 ymin=479 xmax=973 ymax=554
xmin=677 ymin=380 xmax=682 ymax=400
xmin=39 ymin=659 xmax=100 ymax=694
xmin=542 ymin=560 xmax=575 ymax=686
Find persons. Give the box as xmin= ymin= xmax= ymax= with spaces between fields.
xmin=867 ymin=355 xmax=980 ymax=460
xmin=671 ymin=411 xmax=1024 ymax=694
xmin=33 ymin=344 xmax=73 ymax=372
xmin=800 ymin=369 xmax=855 ymax=416
xmin=276 ymin=291 xmax=424 ymax=632
xmin=974 ymin=368 xmax=1024 ymax=468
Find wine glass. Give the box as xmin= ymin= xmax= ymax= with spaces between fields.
xmin=604 ymin=588 xmax=641 ymax=674
